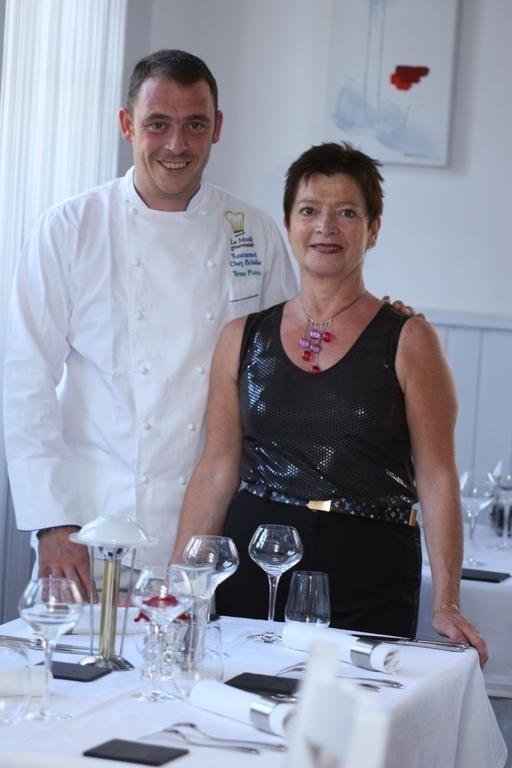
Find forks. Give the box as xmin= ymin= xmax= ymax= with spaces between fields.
xmin=139 ymin=718 xmax=287 ymax=757
xmin=0 ymin=634 xmax=134 ymax=672
xmin=274 ymin=658 xmax=407 ymax=696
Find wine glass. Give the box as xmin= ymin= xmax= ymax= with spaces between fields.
xmin=17 ymin=578 xmax=84 ymax=727
xmin=457 ymin=460 xmax=512 ymax=571
xmin=129 ymin=524 xmax=330 ymax=705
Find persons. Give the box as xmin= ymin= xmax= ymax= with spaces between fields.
xmin=0 ymin=50 xmax=426 ymax=606
xmin=167 ymin=142 xmax=488 ymax=668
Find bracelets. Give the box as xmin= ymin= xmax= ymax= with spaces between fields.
xmin=37 ymin=526 xmax=82 ymax=539
xmin=433 ymin=604 xmax=459 ymax=612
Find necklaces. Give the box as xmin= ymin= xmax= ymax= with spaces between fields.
xmin=298 ymin=290 xmax=366 ymax=375
